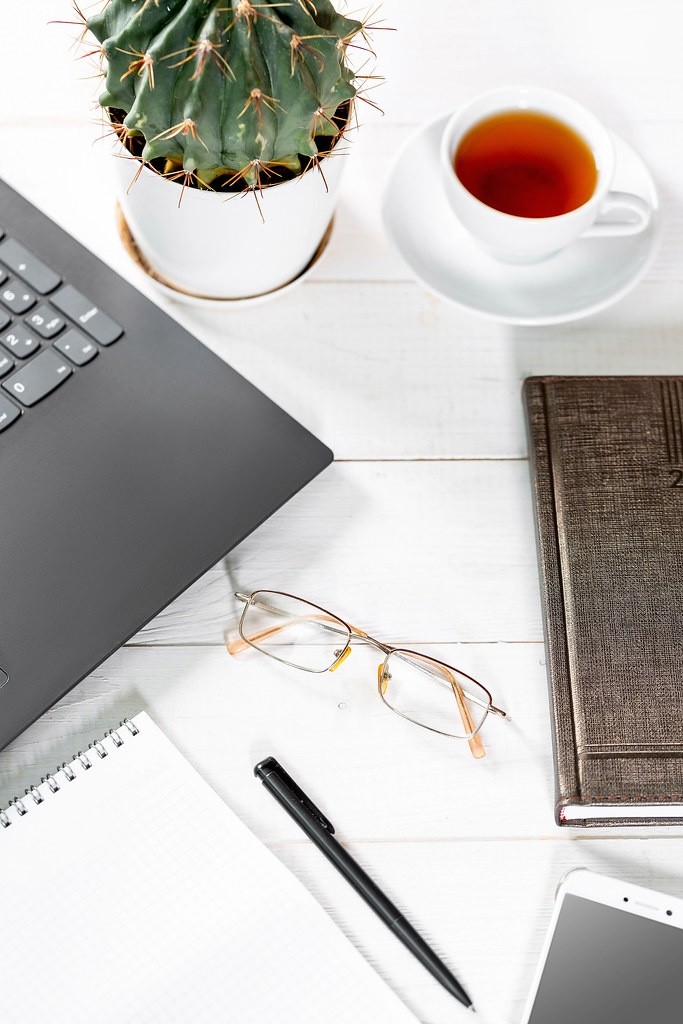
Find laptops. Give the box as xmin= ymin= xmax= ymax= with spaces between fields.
xmin=0 ymin=179 xmax=334 ymax=752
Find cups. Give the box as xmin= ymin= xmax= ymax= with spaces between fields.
xmin=440 ymin=85 xmax=653 ymax=265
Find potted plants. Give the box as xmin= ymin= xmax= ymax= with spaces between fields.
xmin=50 ymin=0 xmax=400 ymax=313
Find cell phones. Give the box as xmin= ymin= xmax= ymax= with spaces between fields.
xmin=520 ymin=865 xmax=683 ymax=1024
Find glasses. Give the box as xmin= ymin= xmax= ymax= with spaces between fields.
xmin=226 ymin=590 xmax=504 ymax=758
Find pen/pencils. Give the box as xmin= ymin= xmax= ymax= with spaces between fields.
xmin=255 ymin=757 xmax=481 ymax=1014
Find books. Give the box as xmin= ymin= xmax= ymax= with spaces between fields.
xmin=0 ymin=712 xmax=427 ymax=1023
xmin=521 ymin=374 xmax=683 ymax=827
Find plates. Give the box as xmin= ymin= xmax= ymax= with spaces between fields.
xmin=380 ymin=111 xmax=665 ymax=326
